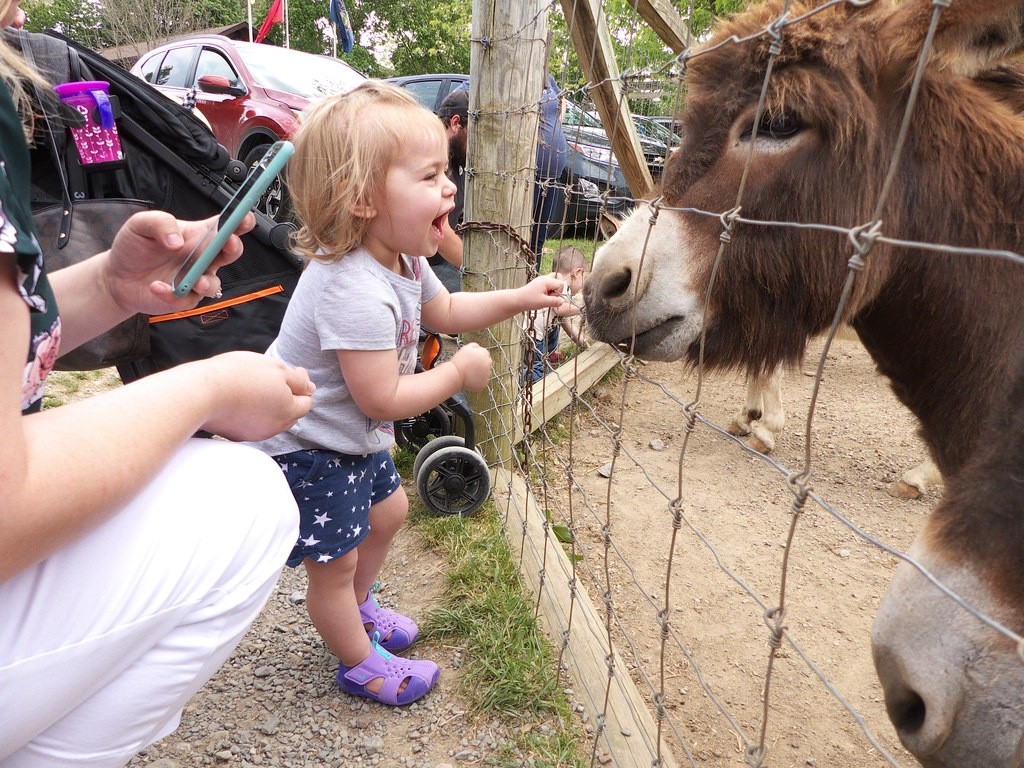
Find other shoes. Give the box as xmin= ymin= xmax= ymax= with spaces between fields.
xmin=550 ymin=352 xmax=568 ymax=362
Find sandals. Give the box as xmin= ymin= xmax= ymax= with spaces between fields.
xmin=357 ymin=592 xmax=419 ymax=655
xmin=336 ymin=631 xmax=439 ymax=706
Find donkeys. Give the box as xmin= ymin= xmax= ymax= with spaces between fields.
xmin=584 ymin=0 xmax=1024 ymax=768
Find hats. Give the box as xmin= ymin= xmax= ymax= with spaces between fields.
xmin=438 ymin=90 xmax=469 ymax=118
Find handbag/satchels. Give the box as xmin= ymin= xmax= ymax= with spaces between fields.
xmin=31 ymin=198 xmax=154 ymax=371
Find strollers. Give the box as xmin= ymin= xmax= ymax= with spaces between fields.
xmin=0 ymin=24 xmax=492 ymax=519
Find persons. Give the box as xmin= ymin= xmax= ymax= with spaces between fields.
xmin=446 ymin=68 xmax=569 ymax=285
xmin=234 ymin=77 xmax=579 ymax=706
xmin=417 ymin=91 xmax=470 ymax=293
xmin=0 ymin=0 xmax=320 ymax=768
xmin=523 ymin=246 xmax=592 ymax=384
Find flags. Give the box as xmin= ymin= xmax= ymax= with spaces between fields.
xmin=254 ymin=0 xmax=283 ymax=44
xmin=329 ymin=0 xmax=354 ymax=54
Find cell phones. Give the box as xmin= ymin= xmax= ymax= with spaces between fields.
xmin=172 ymin=141 xmax=294 ymax=296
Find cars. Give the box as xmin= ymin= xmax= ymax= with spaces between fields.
xmin=379 ymin=74 xmax=682 ymax=241
xmin=128 ymin=33 xmax=373 ymax=224
